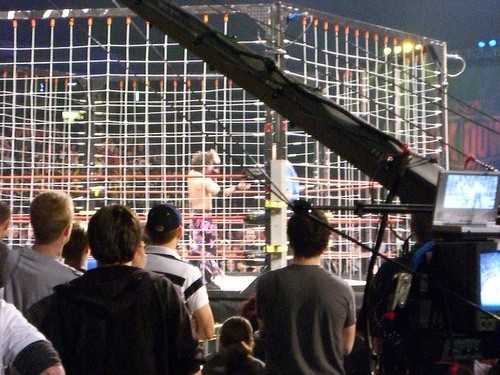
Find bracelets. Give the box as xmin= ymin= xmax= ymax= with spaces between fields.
xmin=234 ymin=187 xmax=239 ymax=191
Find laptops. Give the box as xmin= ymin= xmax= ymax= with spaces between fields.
xmin=431 ymin=171 xmax=500 ymax=234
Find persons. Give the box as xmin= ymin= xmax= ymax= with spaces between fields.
xmin=24 ymin=205 xmax=201 ymax=375
xmin=254 ymin=210 xmax=355 ymax=375
xmin=59 ymin=222 xmax=90 ymax=274
xmin=0 ymin=201 xmax=12 ymax=267
xmin=0 ymin=118 xmax=393 ymax=280
xmin=143 ymin=204 xmax=216 ymax=340
xmin=242 ymin=259 xmax=500 ymax=374
xmin=201 ymin=316 xmax=266 ymax=375
xmin=0 ymin=190 xmax=84 ymax=314
xmin=0 ymin=299 xmax=67 ymax=375
xmin=186 ymin=151 xmax=251 ymax=288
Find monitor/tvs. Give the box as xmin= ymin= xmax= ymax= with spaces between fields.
xmin=473 ymin=243 xmax=500 ymax=333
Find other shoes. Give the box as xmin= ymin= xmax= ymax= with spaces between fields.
xmin=205 ymin=279 xmax=220 ymax=290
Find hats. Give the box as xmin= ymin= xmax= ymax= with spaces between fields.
xmin=147 ymin=203 xmax=183 ymax=231
